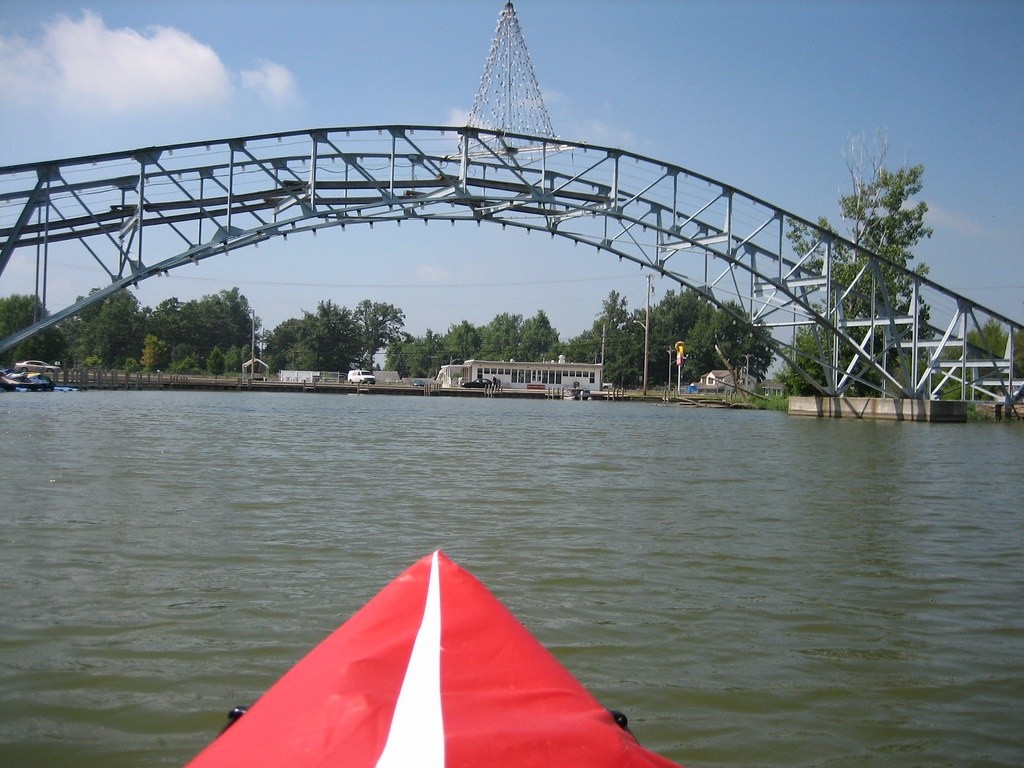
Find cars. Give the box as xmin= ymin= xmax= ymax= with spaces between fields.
xmin=464 ymin=378 xmax=493 ymax=389
xmin=412 ymin=379 xmax=425 ymax=386
xmin=14 ymin=359 xmax=63 ymax=374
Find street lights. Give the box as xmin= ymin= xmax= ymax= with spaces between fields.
xmin=632 ymin=320 xmax=649 ymax=397
xmin=248 ymin=307 xmax=254 ymax=380
xmin=449 ymin=356 xmax=460 ymax=364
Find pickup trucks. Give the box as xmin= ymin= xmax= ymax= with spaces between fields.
xmin=347 ymin=369 xmax=377 ymax=385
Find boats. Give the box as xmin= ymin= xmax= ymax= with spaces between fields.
xmin=0 ymin=368 xmax=56 ymax=392
